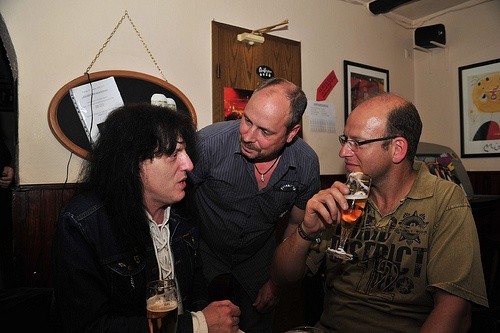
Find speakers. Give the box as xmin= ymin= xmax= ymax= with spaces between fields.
xmin=414 ymin=24 xmax=446 ymax=49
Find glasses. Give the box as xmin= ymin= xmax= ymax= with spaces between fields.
xmin=338 ymin=135 xmax=398 ymax=152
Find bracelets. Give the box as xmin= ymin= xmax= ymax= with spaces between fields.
xmin=297 ymin=224 xmax=323 ymax=243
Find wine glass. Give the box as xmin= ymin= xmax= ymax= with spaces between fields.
xmin=327 ymin=171 xmax=372 ymax=260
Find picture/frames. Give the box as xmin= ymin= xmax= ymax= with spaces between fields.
xmin=343 ymin=60 xmax=389 ymax=131
xmin=458 ymin=58 xmax=500 ymax=158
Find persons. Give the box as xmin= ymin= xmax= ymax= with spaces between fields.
xmin=53 ymin=102 xmax=241 ymax=333
xmin=0 ymin=133 xmax=14 ymax=189
xmin=185 ymin=77 xmax=321 ymax=333
xmin=271 ymin=93 xmax=488 ymax=333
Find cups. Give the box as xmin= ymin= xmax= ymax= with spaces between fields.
xmin=145 ymin=280 xmax=178 ymax=333
